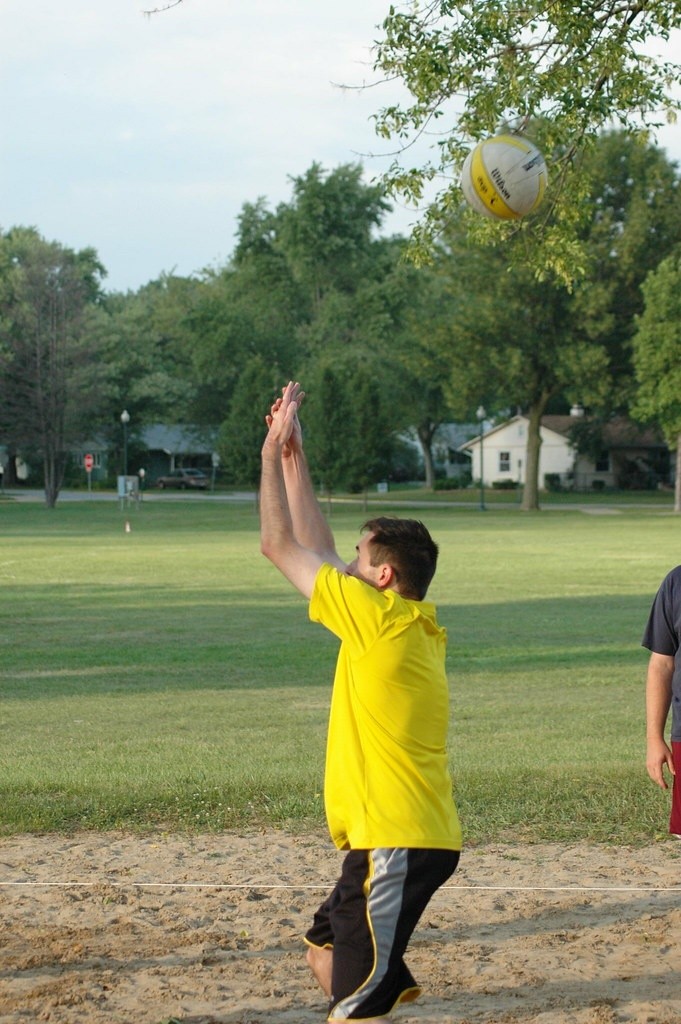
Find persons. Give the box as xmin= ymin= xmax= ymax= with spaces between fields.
xmin=257 ymin=380 xmax=464 ymax=1023
xmin=640 ymin=564 xmax=681 ymax=837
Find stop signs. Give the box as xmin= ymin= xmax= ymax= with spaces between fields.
xmin=84 ymin=454 xmax=93 ymax=472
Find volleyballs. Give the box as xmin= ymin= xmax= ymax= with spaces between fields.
xmin=460 ymin=135 xmax=548 ymax=222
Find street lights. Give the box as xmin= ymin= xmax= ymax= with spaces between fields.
xmin=476 ymin=405 xmax=486 ymax=509
xmin=121 ymin=409 xmax=130 ymax=475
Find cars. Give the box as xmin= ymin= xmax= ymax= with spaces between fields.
xmin=157 ymin=468 xmax=210 ymax=491
